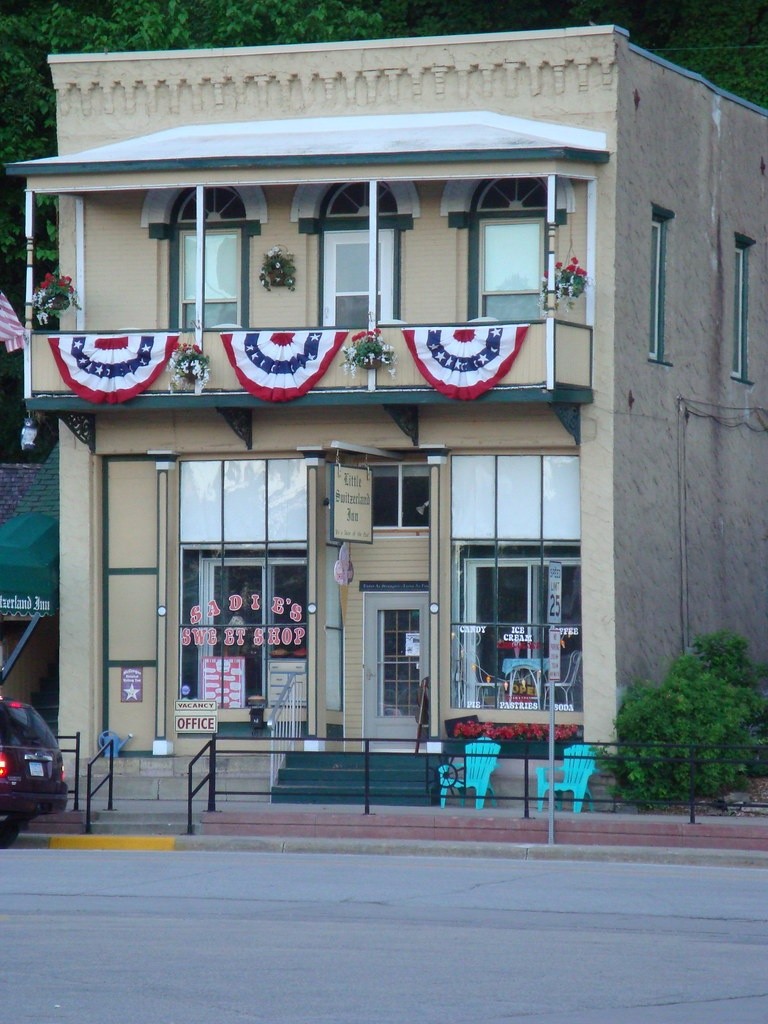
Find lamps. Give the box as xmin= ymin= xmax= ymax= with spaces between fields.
xmin=416 ymin=501 xmax=429 ymax=516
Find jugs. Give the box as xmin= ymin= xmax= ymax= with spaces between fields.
xmin=99 ymin=731 xmax=133 ymax=758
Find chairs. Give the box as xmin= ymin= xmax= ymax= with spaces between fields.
xmin=542 ymin=647 xmax=582 ymax=710
xmin=535 ymin=745 xmax=602 ymax=814
xmin=474 ymin=655 xmax=505 ymax=709
xmin=436 ymin=736 xmax=502 ymax=809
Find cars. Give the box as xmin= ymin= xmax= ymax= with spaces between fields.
xmin=459 ymin=682 xmax=504 ymax=709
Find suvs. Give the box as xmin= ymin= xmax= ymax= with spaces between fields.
xmin=1 ymin=697 xmax=68 ymax=851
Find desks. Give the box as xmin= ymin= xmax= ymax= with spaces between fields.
xmin=503 ymin=657 xmax=549 ymax=710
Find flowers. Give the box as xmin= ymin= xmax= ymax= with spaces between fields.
xmin=166 ymin=342 xmax=215 ymax=395
xmin=338 ymin=328 xmax=399 ymax=379
xmin=453 ymin=719 xmax=578 ymax=741
xmin=259 ymin=247 xmax=297 ymax=292
xmin=541 ymin=257 xmax=588 ymax=314
xmin=32 ymin=273 xmax=82 ymax=325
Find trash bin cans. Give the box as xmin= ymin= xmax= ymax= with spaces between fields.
xmin=248 ymin=694 xmax=266 ymax=736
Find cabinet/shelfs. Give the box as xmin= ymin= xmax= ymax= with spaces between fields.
xmin=267 ymin=659 xmax=307 ymax=708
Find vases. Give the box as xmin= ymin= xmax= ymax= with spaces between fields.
xmin=444 ymin=736 xmax=582 ymax=759
xmin=182 ymin=370 xmax=198 ymax=384
xmin=557 ymin=286 xmax=568 ymax=295
xmin=48 ymin=295 xmax=70 ymax=310
xmin=269 ymin=273 xmax=286 ymax=285
xmin=358 ymin=358 xmax=382 ymax=369
